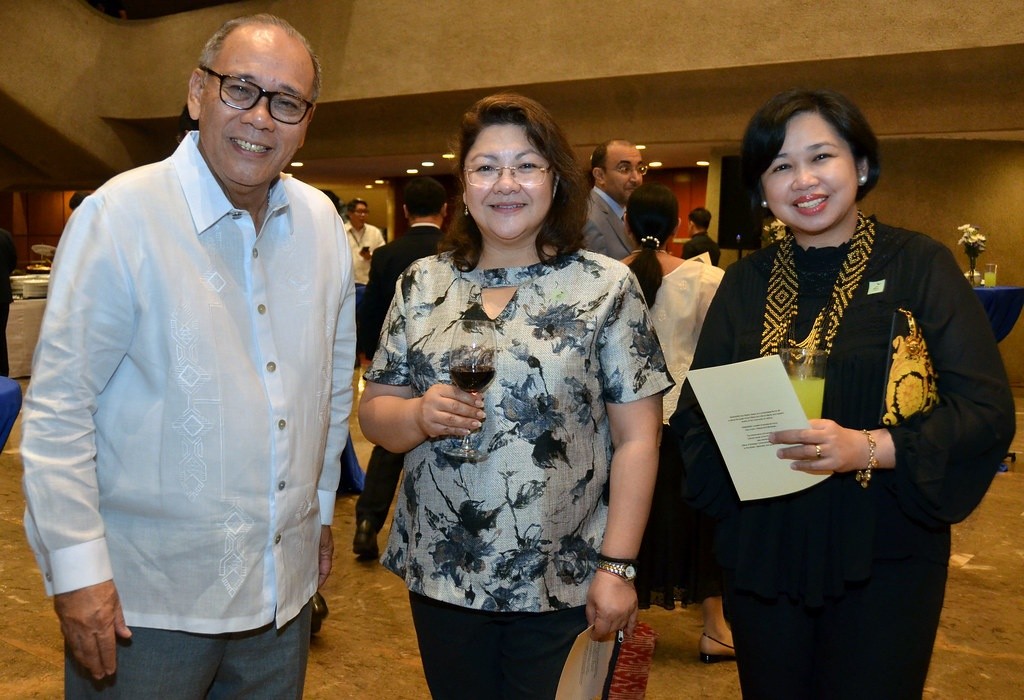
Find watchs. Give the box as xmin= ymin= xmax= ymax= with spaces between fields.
xmin=597 ymin=561 xmax=636 ymax=581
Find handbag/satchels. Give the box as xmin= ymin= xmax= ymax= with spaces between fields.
xmin=555 ymin=613 xmax=656 ymax=700
xmin=874 ymin=308 xmax=942 ymax=427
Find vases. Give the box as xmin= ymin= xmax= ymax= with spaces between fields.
xmin=967 ymin=254 xmax=978 ymax=290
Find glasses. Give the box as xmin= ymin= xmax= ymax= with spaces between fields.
xmin=354 ymin=209 xmax=369 ymax=215
xmin=606 ymin=165 xmax=647 ymax=177
xmin=462 ymin=164 xmax=552 ymax=187
xmin=200 ymin=64 xmax=313 ymax=125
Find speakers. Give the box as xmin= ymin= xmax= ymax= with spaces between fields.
xmin=717 ymin=154 xmax=762 ymax=250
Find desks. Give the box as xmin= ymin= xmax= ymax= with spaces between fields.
xmin=973 ymin=286 xmax=1024 ymax=344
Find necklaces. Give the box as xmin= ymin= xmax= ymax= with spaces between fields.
xmin=760 ymin=209 xmax=874 ymax=378
xmin=349 ymin=229 xmax=365 ymax=247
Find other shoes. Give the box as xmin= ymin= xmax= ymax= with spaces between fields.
xmin=698 ymin=632 xmax=736 ymax=663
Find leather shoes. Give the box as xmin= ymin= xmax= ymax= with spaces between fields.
xmin=353 ymin=518 xmax=378 ymax=558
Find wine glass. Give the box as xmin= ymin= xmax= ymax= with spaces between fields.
xmin=440 ymin=318 xmax=498 ymax=459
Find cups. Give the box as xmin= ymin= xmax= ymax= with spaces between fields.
xmin=984 ymin=263 xmax=997 ymax=288
xmin=779 ymin=347 xmax=827 ymax=422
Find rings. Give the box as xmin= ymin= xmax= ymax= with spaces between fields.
xmin=816 ymin=444 xmax=823 ymax=459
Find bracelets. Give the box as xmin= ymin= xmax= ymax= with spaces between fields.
xmin=598 ymin=553 xmax=637 ymax=563
xmin=856 ymin=428 xmax=879 ymax=488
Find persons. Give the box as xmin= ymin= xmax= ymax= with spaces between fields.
xmin=581 ymin=138 xmax=649 ymax=260
xmin=353 ymin=177 xmax=447 ymax=558
xmin=68 ymin=191 xmax=90 ymax=210
xmin=357 ymin=91 xmax=676 ymax=700
xmin=679 ymin=88 xmax=1017 ymax=699
xmin=617 ymin=184 xmax=736 ymax=665
xmin=343 ymin=199 xmax=387 ymax=369
xmin=17 ymin=13 xmax=356 ymax=700
xmin=682 ymin=207 xmax=721 ymax=266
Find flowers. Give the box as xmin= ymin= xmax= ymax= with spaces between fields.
xmin=956 ymin=220 xmax=984 ymax=265
